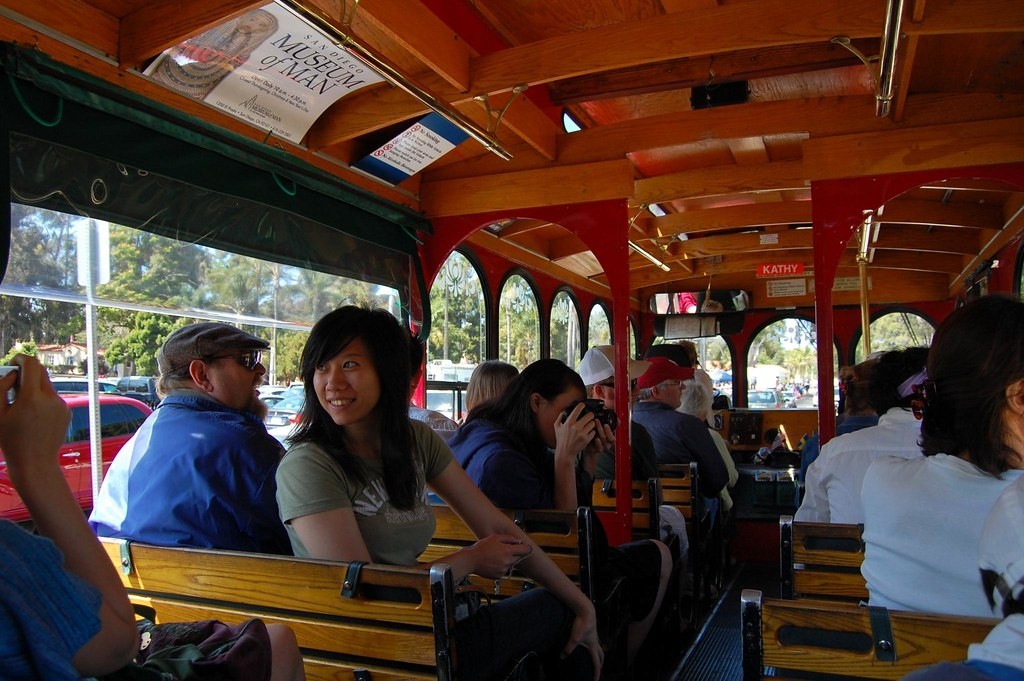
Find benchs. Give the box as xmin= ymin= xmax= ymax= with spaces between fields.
xmin=740 ymin=590 xmax=1005 ymax=681
xmin=781 ymin=516 xmax=867 ymax=604
xmin=413 ymin=503 xmax=631 ymax=681
xmin=95 ymin=538 xmax=545 ymax=681
xmin=661 ymin=461 xmax=722 ymax=611
xmin=593 ymin=480 xmax=678 ymax=663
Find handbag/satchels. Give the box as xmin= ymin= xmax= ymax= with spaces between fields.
xmin=94 ymin=618 xmax=272 ymax=681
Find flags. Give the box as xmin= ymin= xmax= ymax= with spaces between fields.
xmin=772 ymin=432 xmax=785 ymax=451
xmin=799 ymin=434 xmax=810 ymax=450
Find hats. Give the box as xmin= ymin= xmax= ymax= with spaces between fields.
xmin=157 ymin=321 xmax=271 ymax=376
xmin=575 ymin=345 xmax=652 ymax=386
xmin=637 ymin=357 xmax=695 ymax=389
xmin=645 ymin=344 xmax=692 ymax=367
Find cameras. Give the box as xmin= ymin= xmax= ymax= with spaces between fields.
xmin=0 ymin=365 xmax=20 ymax=404
xmin=562 ymin=399 xmax=617 ymax=439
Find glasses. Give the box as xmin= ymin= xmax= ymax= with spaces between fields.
xmin=660 ymin=381 xmax=681 ymax=387
xmin=204 ymin=348 xmax=262 ymax=371
xmin=600 ymin=378 xmax=637 ymax=391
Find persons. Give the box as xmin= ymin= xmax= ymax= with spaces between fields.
xmin=274 ymin=305 xmax=607 ymax=681
xmin=424 ymin=360 xmax=676 ymax=681
xmin=0 ymin=353 xmax=311 ymax=681
xmin=675 ymin=291 xmax=737 ymax=313
xmin=792 ymin=294 xmax=1024 ymax=680
xmin=397 ymin=325 xmax=461 ymax=443
xmin=572 ymin=337 xmax=742 ymax=641
xmin=455 ymin=360 xmax=520 ymax=428
xmin=87 ymin=322 xmax=296 ymax=557
xmin=711 ymin=367 xmax=811 ymax=409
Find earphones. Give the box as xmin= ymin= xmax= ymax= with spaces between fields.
xmin=534 ymin=398 xmax=540 ymax=404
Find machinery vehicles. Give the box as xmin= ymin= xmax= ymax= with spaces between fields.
xmin=427 ymin=357 xmax=477 ymax=382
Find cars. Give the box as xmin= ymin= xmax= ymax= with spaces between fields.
xmin=1 ymin=392 xmax=155 ymax=523
xmin=115 ymin=377 xmax=159 ymax=408
xmin=48 ymin=376 xmax=117 ymax=394
xmin=257 ymin=382 xmax=307 ymax=428
xmin=748 ymin=381 xmax=808 ymax=410
